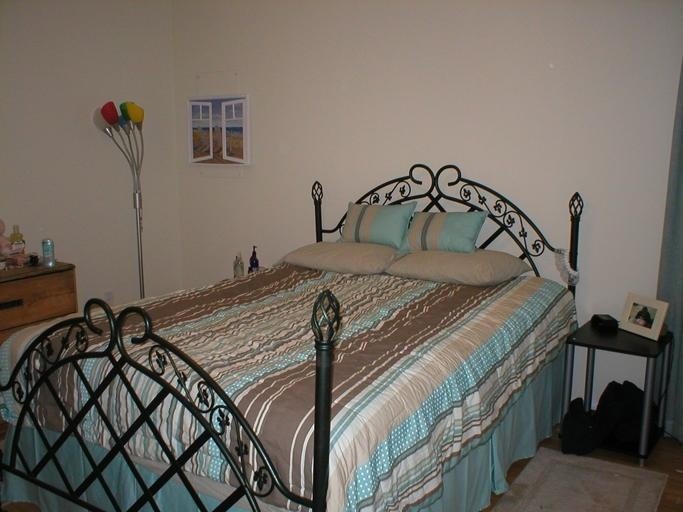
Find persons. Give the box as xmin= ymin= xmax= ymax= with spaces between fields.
xmin=633 ymin=306 xmax=651 ymax=327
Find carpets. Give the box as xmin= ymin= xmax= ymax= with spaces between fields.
xmin=492 ymin=447 xmax=670 ymax=512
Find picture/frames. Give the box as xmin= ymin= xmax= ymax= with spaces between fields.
xmin=616 ymin=293 xmax=669 ymax=342
xmin=186 ymin=96 xmax=251 ymax=166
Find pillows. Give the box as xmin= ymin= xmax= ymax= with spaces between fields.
xmin=285 ymin=239 xmax=394 ymax=276
xmin=407 ymin=211 xmax=487 ymax=253
xmin=341 ymin=200 xmax=415 ymax=247
xmin=387 ymin=236 xmax=528 ymax=287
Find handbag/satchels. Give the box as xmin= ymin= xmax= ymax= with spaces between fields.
xmin=558 ymin=397 xmax=597 ymax=455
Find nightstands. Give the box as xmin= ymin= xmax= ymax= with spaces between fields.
xmin=0 ymin=254 xmax=76 ymax=344
xmin=561 ymin=316 xmax=673 ymax=469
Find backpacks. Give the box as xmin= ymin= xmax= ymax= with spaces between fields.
xmin=592 ymin=380 xmax=659 ymax=453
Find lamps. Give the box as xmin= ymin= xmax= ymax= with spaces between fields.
xmin=91 ymin=98 xmax=145 ymax=302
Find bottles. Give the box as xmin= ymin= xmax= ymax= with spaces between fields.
xmin=251 ymin=251 xmax=258 ymax=272
xmin=247 ymin=267 xmax=252 ymax=274
xmin=10 ymin=225 xmax=24 ymax=254
xmin=234 ymin=255 xmax=244 ymax=278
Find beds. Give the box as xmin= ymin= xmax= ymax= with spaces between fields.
xmin=1 ymin=163 xmax=584 ymax=512
